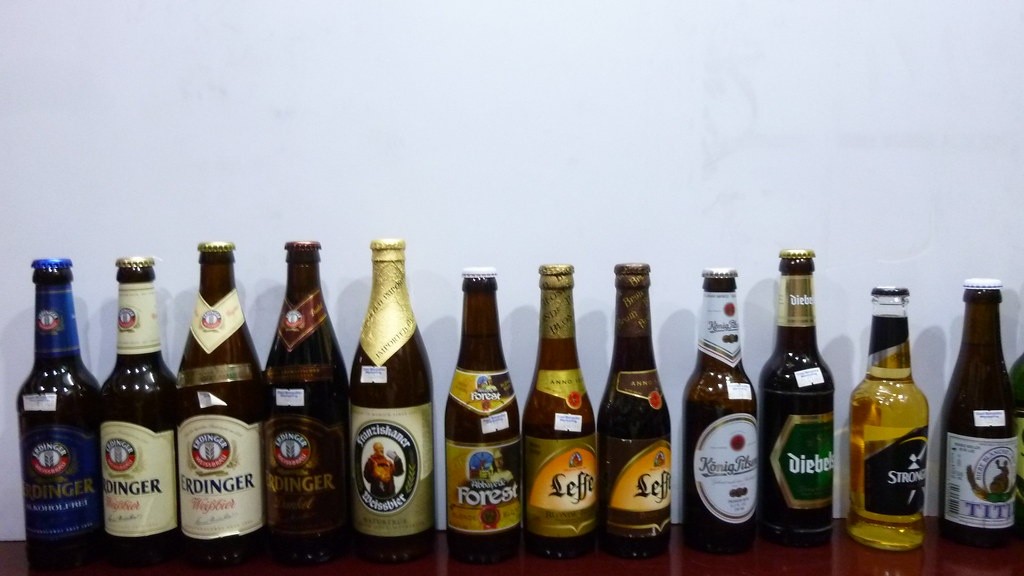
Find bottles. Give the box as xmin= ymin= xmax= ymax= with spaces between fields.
xmin=94 ymin=258 xmax=179 ymax=567
xmin=937 ymin=279 xmax=1021 ymax=549
xmin=349 ymin=239 xmax=437 ymax=567
xmin=16 ymin=258 xmax=104 ymax=570
xmin=1009 ymin=353 xmax=1024 ymax=541
xmin=759 ymin=250 xmax=835 ymax=547
xmin=847 ymin=286 xmax=929 ymax=552
xmin=265 ymin=245 xmax=349 ymax=567
xmin=178 ymin=246 xmax=265 ymax=569
xmin=598 ymin=261 xmax=671 ymax=561
xmin=444 ymin=268 xmax=522 ymax=563
xmin=522 ymin=265 xmax=598 ymax=558
xmin=682 ymin=268 xmax=759 ymax=553
